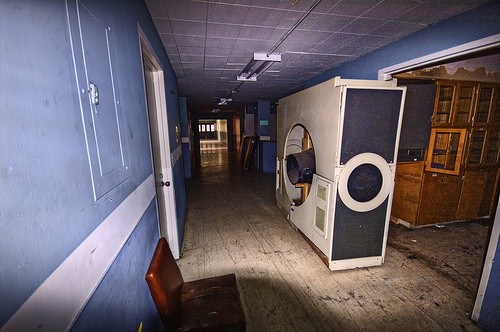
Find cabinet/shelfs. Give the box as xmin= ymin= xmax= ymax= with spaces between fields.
xmin=391 ymin=76 xmax=500 ymax=229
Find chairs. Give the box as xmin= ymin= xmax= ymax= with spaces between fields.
xmin=146 ymin=237 xmax=247 ymax=332
